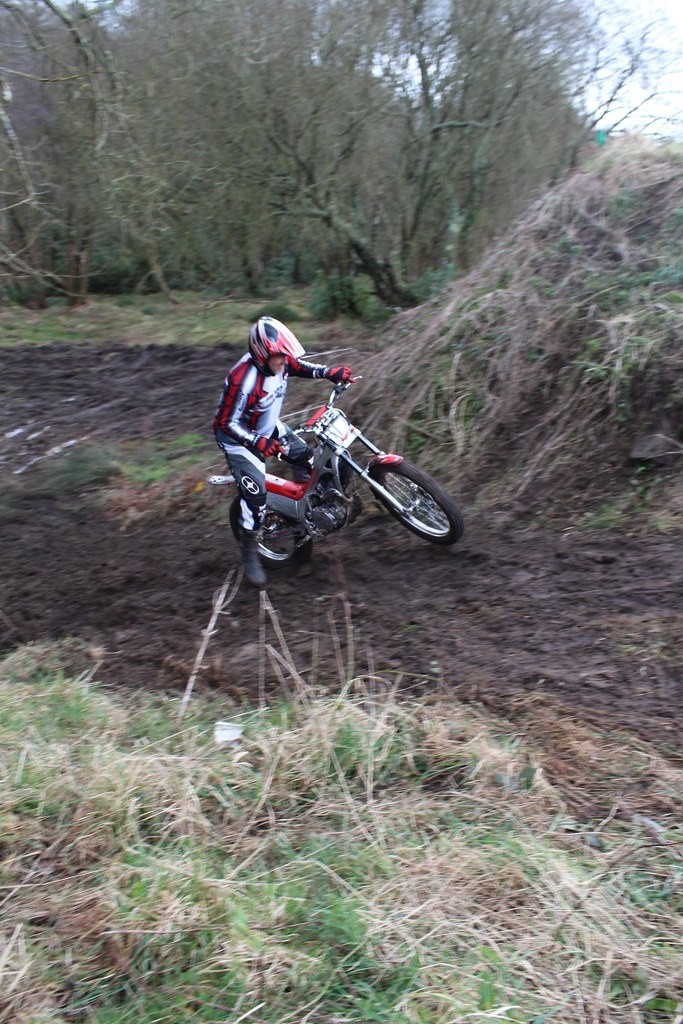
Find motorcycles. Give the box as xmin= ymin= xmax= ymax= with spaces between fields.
xmin=205 ymin=374 xmax=466 ymax=571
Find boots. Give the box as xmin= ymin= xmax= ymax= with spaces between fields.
xmin=238 ymin=519 xmax=267 ymax=586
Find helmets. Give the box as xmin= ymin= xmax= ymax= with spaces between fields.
xmin=249 ymin=316 xmax=306 ymax=377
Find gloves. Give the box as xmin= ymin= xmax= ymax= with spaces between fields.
xmin=328 ymin=364 xmax=357 ymax=384
xmin=254 ymin=437 xmax=285 ymax=457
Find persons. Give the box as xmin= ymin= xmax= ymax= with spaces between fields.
xmin=211 ymin=316 xmax=357 ymax=584
